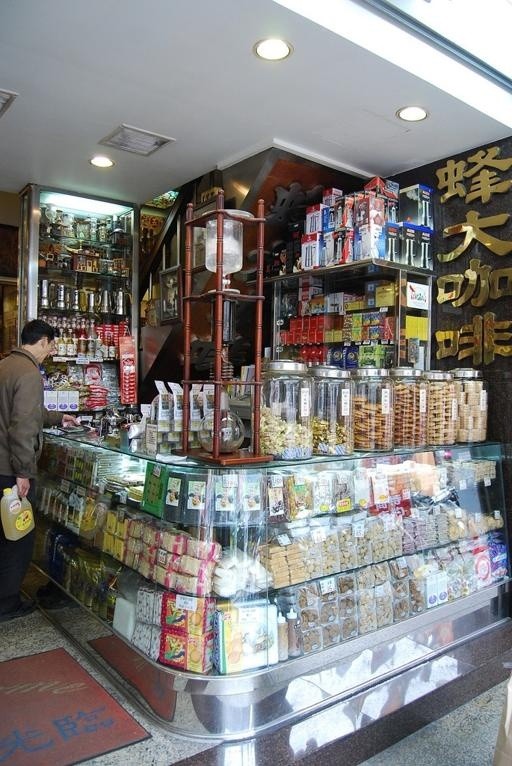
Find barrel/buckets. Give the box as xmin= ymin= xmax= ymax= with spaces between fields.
xmin=0 ymin=484 xmax=35 ymax=541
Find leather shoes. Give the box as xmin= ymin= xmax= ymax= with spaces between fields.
xmin=0 ymin=602 xmax=38 ymax=622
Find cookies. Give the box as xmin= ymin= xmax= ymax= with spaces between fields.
xmin=353 ymin=379 xmax=488 ymax=450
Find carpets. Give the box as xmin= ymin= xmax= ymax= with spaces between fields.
xmin=0 ymin=648 xmax=153 ymax=766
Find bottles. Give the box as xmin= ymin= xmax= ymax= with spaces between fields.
xmin=356 ymin=363 xmax=396 ymax=453
xmin=309 ymin=361 xmax=356 ymax=457
xmin=423 ymin=366 xmax=460 ymax=446
xmin=38 ymin=314 xmax=118 ymax=358
xmin=197 ymin=392 xmax=247 ymax=454
xmin=249 ymin=357 xmax=314 ymax=462
xmin=390 ymin=364 xmax=431 ymax=452
xmin=40 ymin=276 xmax=133 ymax=316
xmin=448 ymin=367 xmax=489 ymax=443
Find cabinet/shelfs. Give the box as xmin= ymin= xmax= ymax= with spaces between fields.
xmin=16 ymin=181 xmax=511 ymax=743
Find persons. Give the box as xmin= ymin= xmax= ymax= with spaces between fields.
xmin=0 ymin=318 xmax=81 ymax=623
